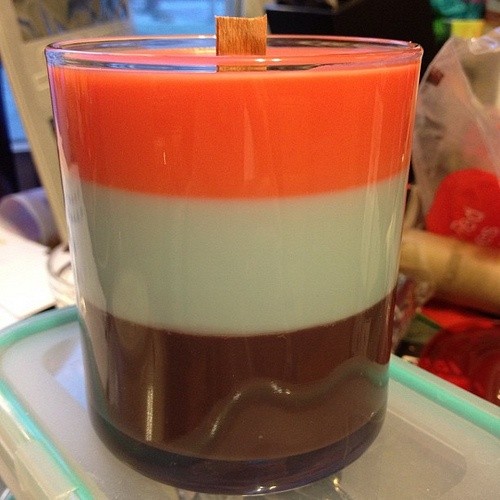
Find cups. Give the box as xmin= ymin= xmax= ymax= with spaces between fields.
xmin=44 ymin=35 xmax=424 ymax=496
